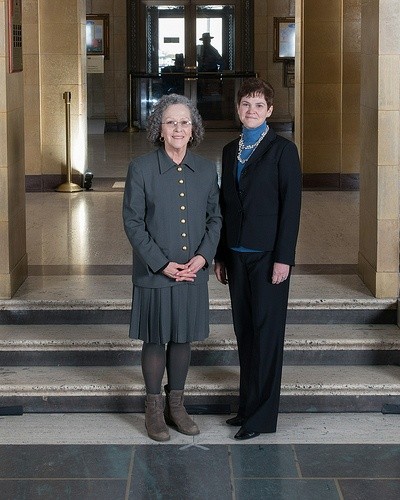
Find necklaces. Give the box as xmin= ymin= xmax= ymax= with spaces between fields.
xmin=237 ymin=127 xmax=269 ymax=165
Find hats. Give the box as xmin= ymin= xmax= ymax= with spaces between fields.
xmin=171 ymin=53 xmax=185 ymax=62
xmin=199 ymin=33 xmax=214 ymax=41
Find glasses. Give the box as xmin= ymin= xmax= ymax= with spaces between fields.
xmin=158 ymin=120 xmax=193 ymax=127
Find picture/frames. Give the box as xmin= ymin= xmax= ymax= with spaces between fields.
xmin=85 ymin=13 xmax=112 ymax=60
xmin=272 ymin=16 xmax=296 ymax=64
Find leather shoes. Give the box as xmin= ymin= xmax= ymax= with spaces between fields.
xmin=226 ymin=416 xmax=261 ymax=441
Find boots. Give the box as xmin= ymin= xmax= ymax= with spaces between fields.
xmin=144 ymin=390 xmax=200 ymax=442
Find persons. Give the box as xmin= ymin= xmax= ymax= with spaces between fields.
xmin=123 ymin=95 xmax=221 ymax=442
xmin=162 ymin=33 xmax=226 ymax=127
xmin=215 ymin=77 xmax=301 ymax=439
